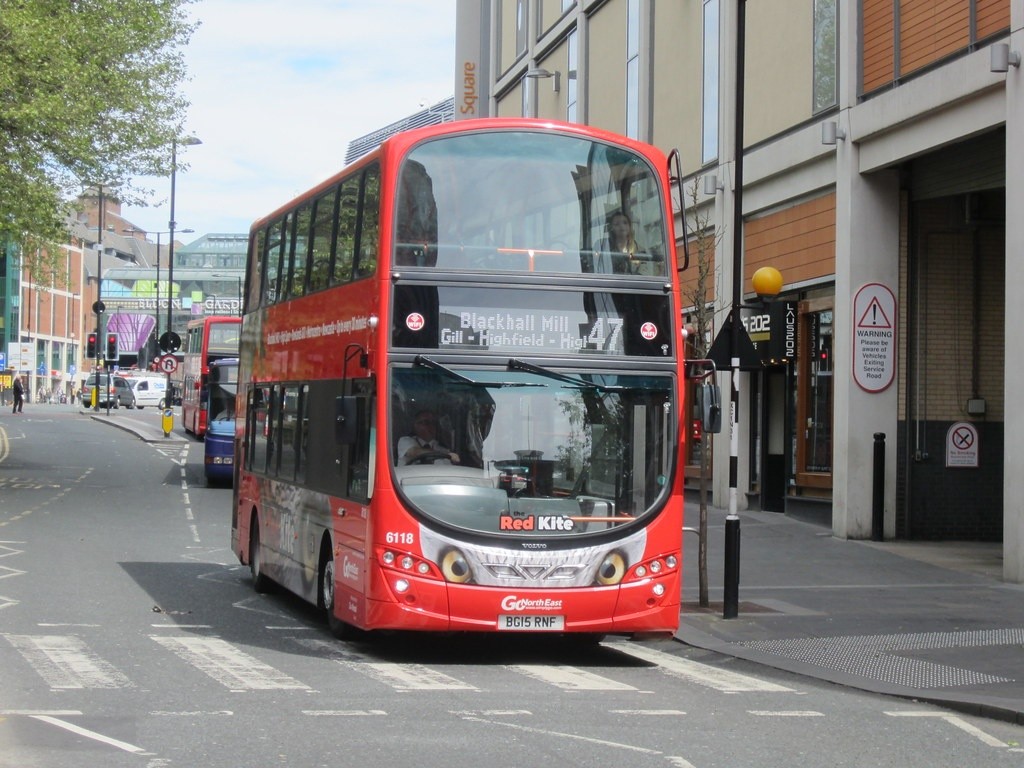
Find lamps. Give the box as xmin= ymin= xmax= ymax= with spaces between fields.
xmin=705 ymin=175 xmax=724 ymax=194
xmin=991 ymin=42 xmax=1020 ymax=72
xmin=821 ymin=121 xmax=844 ymax=145
xmin=526 ymin=68 xmax=561 ymax=91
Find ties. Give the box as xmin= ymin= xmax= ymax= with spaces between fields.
xmin=425 ymin=444 xmax=431 ymax=450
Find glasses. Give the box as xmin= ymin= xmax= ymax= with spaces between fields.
xmin=415 ymin=419 xmax=437 ymax=426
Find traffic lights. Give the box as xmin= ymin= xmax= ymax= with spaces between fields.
xmin=105 ymin=332 xmax=119 ymax=362
xmin=820 ymin=348 xmax=828 ymax=371
xmin=84 ymin=333 xmax=97 ymax=359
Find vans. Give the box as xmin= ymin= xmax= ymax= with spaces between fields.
xmin=124 ymin=377 xmax=168 ymax=410
xmin=82 ymin=372 xmax=136 ymax=409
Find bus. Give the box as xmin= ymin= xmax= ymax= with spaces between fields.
xmin=98 ymin=350 xmax=185 ymax=390
xmin=231 ymin=119 xmax=721 ymax=650
xmin=204 ymin=356 xmax=242 ymax=486
xmin=181 ymin=314 xmax=243 ymax=442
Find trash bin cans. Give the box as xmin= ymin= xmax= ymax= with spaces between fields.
xmin=514 ymin=449 xmax=544 ymax=460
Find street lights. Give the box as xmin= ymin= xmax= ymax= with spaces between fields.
xmin=71 ymin=293 xmax=80 ymax=405
xmin=209 ymin=294 xmax=216 ymax=314
xmin=163 ymin=135 xmax=204 ymax=437
xmin=120 ymin=228 xmax=195 ymax=372
xmin=212 ymin=274 xmax=242 ymax=318
xmin=80 ymin=178 xmax=123 ymax=413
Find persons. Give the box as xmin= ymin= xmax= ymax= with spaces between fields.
xmin=38 ymin=386 xmax=52 ymax=406
xmin=215 ymin=400 xmax=236 ymax=421
xmin=56 ymin=385 xmax=63 ymax=406
xmin=76 ymin=388 xmax=82 ymax=406
xmin=398 ymin=410 xmax=462 ymax=466
xmin=13 ymin=375 xmax=24 ymax=414
xmin=592 ymin=212 xmax=661 ymax=356
